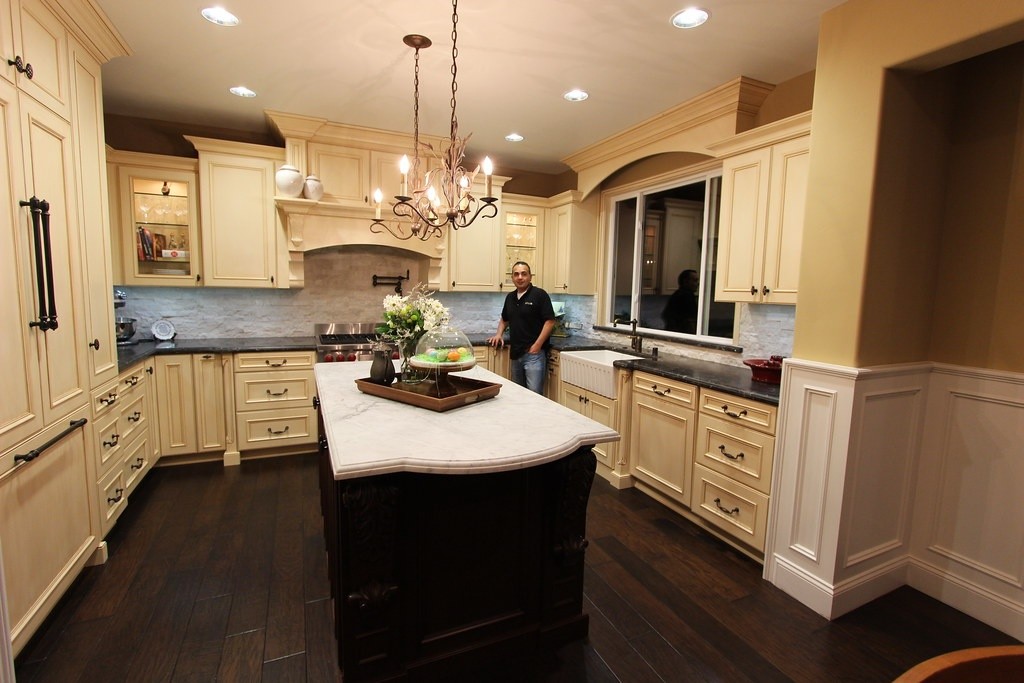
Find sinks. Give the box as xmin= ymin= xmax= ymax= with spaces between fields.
xmin=559 ymin=349 xmax=645 ymax=399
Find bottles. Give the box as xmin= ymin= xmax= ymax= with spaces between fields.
xmin=162 ymin=182 xmax=170 ymax=195
xmin=304 ymin=174 xmax=325 ymax=201
xmin=276 ymin=164 xmax=303 ymax=198
xmin=168 ymin=233 xmax=185 ymax=250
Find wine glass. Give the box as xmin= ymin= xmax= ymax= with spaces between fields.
xmin=505 ymin=215 xmax=534 ymax=273
xmin=139 ymin=197 xmax=187 ymax=223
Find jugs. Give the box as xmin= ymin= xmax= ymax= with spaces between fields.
xmin=370 ymin=349 xmax=396 ymax=385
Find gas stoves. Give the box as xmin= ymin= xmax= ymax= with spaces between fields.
xmin=313 ymin=322 xmax=386 ymax=361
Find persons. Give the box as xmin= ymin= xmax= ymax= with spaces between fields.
xmin=661 ymin=270 xmax=699 ymax=337
xmin=486 ymin=261 xmax=556 ymax=397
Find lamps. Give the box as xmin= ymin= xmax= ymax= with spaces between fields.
xmin=391 ymin=0 xmax=499 ymax=233
xmin=369 ymin=34 xmax=442 ymax=242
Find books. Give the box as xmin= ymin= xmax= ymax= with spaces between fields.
xmin=136 ymin=226 xmax=166 ymax=260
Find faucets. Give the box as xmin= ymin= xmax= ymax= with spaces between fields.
xmin=613 ymin=319 xmax=643 ymax=353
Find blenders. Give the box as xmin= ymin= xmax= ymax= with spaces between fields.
xmin=114 ymin=290 xmax=139 ymax=345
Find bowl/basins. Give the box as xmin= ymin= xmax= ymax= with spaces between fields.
xmin=115 ymin=318 xmax=137 ymax=341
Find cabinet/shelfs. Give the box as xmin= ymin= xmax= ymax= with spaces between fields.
xmin=314 ymin=360 xmax=622 ymax=683
xmin=0 ymin=0 xmax=813 ymax=683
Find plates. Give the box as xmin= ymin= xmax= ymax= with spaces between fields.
xmin=151 ymin=319 xmax=175 ymax=340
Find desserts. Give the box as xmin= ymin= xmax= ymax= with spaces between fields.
xmin=426 ymin=347 xmax=472 ymax=361
